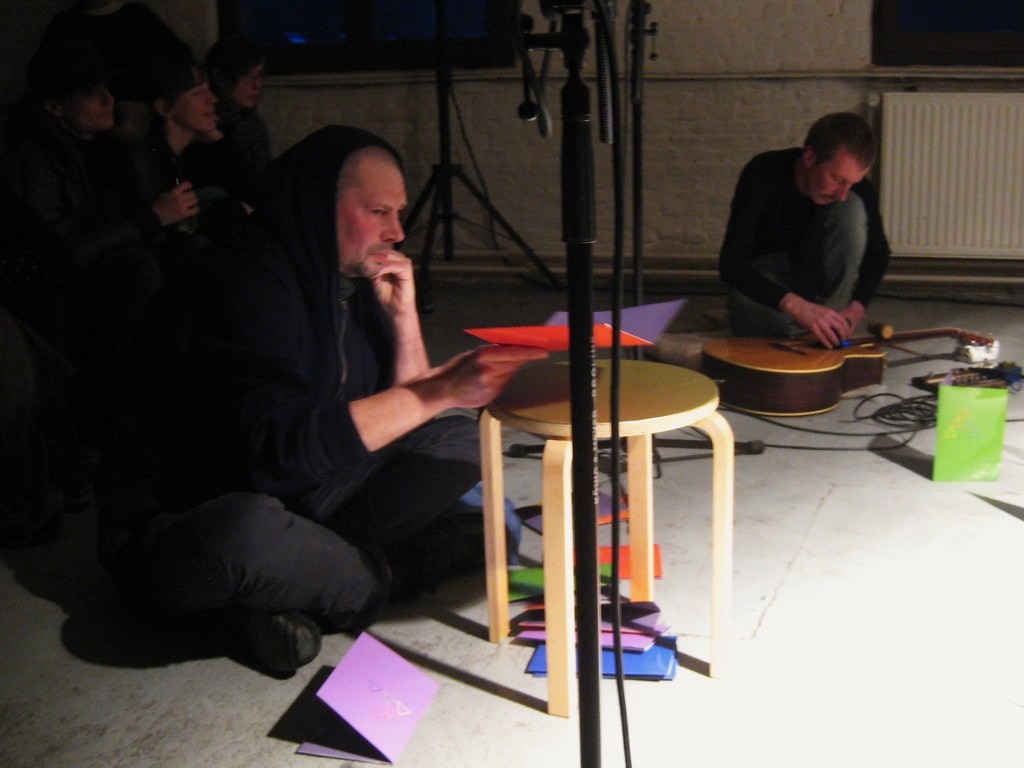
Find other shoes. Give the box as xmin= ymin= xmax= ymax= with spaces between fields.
xmin=393 ymin=506 xmax=517 ymax=598
xmin=238 ymin=605 xmax=321 ymax=670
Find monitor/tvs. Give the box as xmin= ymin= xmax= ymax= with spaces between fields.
xmin=872 ymin=1 xmax=1024 ymax=69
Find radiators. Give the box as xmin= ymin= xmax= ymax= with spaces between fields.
xmin=866 ymin=91 xmax=1024 ymax=286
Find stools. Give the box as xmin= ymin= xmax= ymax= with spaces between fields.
xmin=476 ymin=358 xmax=736 ymax=720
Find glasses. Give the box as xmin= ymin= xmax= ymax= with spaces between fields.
xmin=235 ymin=70 xmax=267 ymax=85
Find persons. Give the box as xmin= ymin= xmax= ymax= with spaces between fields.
xmin=28 ymin=0 xmax=196 ymax=61
xmin=718 ymin=112 xmax=892 ymax=351
xmin=96 ymin=126 xmax=550 ymax=674
xmin=0 ymin=39 xmax=275 ymax=513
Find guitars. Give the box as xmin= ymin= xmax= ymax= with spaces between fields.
xmin=700 ymin=325 xmax=995 ymax=419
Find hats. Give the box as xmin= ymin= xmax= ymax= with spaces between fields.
xmin=30 ymin=40 xmax=119 ymax=99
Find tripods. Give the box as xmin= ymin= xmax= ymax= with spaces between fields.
xmin=390 ymin=1 xmax=564 ymax=313
